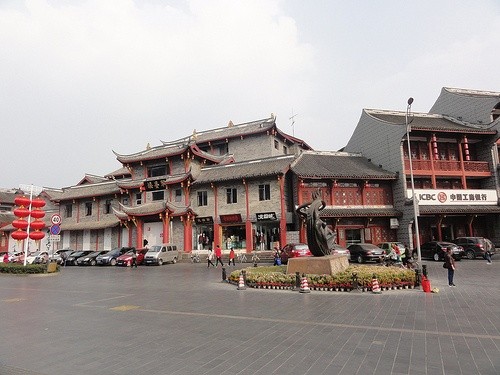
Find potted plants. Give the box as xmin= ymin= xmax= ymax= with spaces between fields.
xmin=224 ymin=266 xmax=416 ymax=292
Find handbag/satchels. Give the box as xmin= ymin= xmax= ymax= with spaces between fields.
xmin=443 ymin=263 xmax=448 ymax=268
xmin=397 ymin=255 xmax=402 ymax=262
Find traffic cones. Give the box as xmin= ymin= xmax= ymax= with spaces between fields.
xmin=238 ymin=273 xmax=246 ymax=290
xmin=299 ymin=273 xmax=311 ymax=293
xmin=372 ymin=274 xmax=381 ymax=294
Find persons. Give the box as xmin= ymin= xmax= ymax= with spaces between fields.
xmin=3 ymin=252 xmax=9 ymax=263
xmin=227 ymin=248 xmax=235 ymax=267
xmin=273 ymin=247 xmax=288 ymax=266
xmin=482 ymin=237 xmax=492 ymax=265
xmin=207 ymin=245 xmax=225 ymax=267
xmin=19 ymin=251 xmax=24 ymax=262
xmin=60 ymin=251 xmax=67 ymax=268
xmin=131 ymin=250 xmax=137 ymax=268
xmin=444 ymin=247 xmax=456 ymax=287
xmin=256 ymin=231 xmax=265 ymax=252
xmin=44 ymin=252 xmax=49 ymax=263
xmin=385 ymin=243 xmax=411 ymax=267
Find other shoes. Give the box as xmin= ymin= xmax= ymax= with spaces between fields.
xmin=450 ymin=284 xmax=456 ymax=287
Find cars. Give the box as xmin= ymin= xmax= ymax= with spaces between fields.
xmin=412 ymin=241 xmax=465 ymax=262
xmin=376 ymin=241 xmax=406 ymax=261
xmin=0 ymin=248 xmax=109 ymax=266
xmin=331 ymin=243 xmax=387 ymax=263
xmin=115 ymin=248 xmax=149 ymax=267
xmin=273 ymin=242 xmax=314 ymax=263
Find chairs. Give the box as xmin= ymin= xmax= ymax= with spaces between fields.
xmin=154 ymin=248 xmax=157 ymax=251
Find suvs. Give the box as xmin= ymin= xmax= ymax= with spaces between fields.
xmin=96 ymin=246 xmax=136 ymax=265
xmin=451 ymin=236 xmax=496 ymax=260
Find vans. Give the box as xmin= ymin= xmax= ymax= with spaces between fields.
xmin=144 ymin=243 xmax=179 ymax=266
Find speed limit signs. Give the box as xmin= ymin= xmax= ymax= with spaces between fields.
xmin=51 ymin=213 xmax=62 ymax=225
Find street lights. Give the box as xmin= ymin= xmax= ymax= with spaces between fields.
xmin=406 ymin=97 xmax=422 ymax=272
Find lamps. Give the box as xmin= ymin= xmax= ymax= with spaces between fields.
xmin=365 ymin=180 xmax=370 ymax=189
xmin=300 ymin=180 xmax=305 ymax=187
xmin=333 ymin=180 xmax=338 ymax=187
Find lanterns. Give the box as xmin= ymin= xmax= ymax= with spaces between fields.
xmin=11 ymin=195 xmax=46 ymax=243
